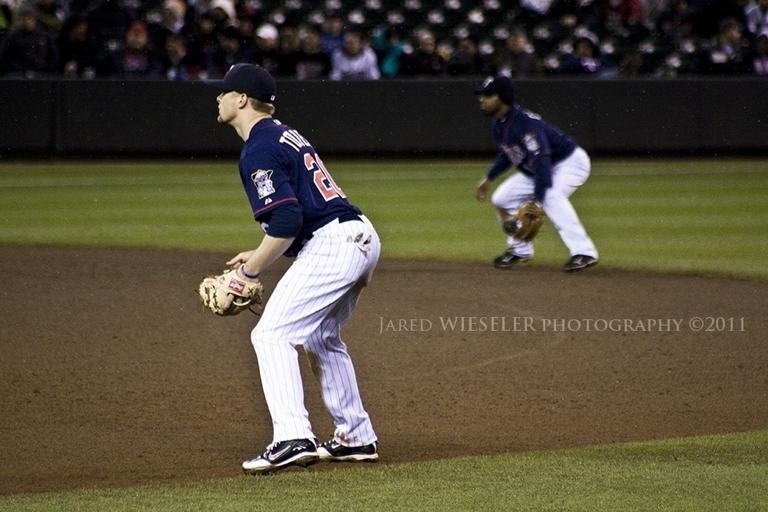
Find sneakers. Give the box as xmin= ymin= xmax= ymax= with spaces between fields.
xmin=563 ymin=251 xmax=599 ymax=272
xmin=239 ymin=436 xmax=321 ymax=474
xmin=493 ymin=248 xmax=536 ymax=271
xmin=316 ymin=434 xmax=381 ymax=462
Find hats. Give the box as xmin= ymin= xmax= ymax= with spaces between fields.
xmin=471 ymin=75 xmax=516 ymax=99
xmin=201 ymin=62 xmax=279 ymax=104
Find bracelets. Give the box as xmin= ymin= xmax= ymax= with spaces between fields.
xmin=240 ymin=265 xmax=260 ymax=278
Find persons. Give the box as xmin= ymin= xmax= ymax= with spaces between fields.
xmin=199 ymin=63 xmax=381 ymax=473
xmin=476 ymin=77 xmax=600 ymax=271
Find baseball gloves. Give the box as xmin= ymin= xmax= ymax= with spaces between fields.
xmin=503 ymin=201 xmax=544 ymax=243
xmin=199 ymin=264 xmax=264 ymax=316
